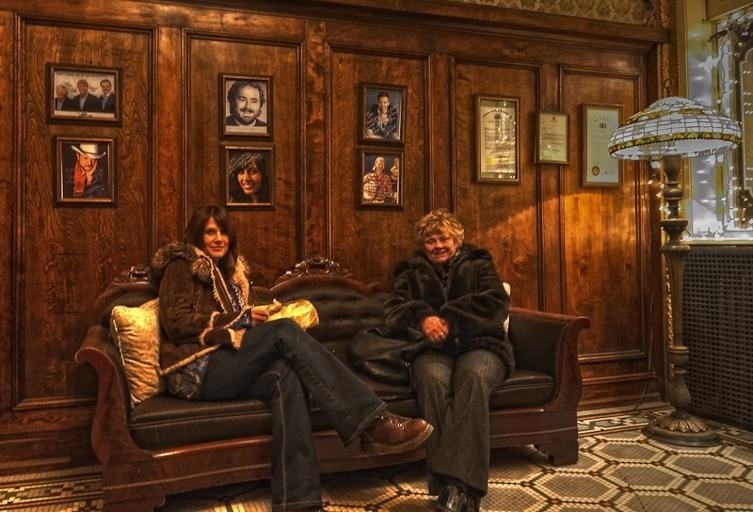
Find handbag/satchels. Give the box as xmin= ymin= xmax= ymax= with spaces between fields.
xmin=349 ymin=327 xmax=429 ymax=385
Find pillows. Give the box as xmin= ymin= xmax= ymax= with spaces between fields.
xmin=104 ymin=295 xmax=166 ymax=408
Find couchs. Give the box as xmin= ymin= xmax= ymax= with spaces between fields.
xmin=66 ymin=253 xmax=595 ymax=512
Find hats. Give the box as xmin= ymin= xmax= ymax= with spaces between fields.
xmin=72 ymin=143 xmax=107 ymax=159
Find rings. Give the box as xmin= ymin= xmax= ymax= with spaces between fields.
xmin=440 ymin=332 xmax=443 ymax=337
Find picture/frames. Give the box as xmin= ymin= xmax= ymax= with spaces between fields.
xmin=353 ymin=144 xmax=403 ymax=211
xmin=473 ymin=92 xmax=522 ymax=185
xmin=357 ymin=81 xmax=407 ymax=147
xmin=46 ymin=60 xmax=124 ymax=127
xmin=219 ymin=141 xmax=276 ymax=211
xmin=52 ymin=133 xmax=118 ymax=208
xmin=218 ymin=71 xmax=275 ymax=143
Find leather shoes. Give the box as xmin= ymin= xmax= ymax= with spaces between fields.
xmin=359 ymin=413 xmax=433 ymax=455
xmin=437 ymin=485 xmax=481 ymax=512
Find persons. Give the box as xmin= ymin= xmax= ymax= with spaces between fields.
xmin=64 ymin=143 xmax=108 ymax=199
xmin=99 ymin=79 xmax=114 ymax=112
xmin=55 ymin=84 xmax=77 ymax=111
xmin=149 ymin=202 xmax=435 ymax=512
xmin=73 ymin=79 xmax=99 ymax=111
xmin=365 ymin=92 xmax=397 ymax=140
xmin=383 ymin=208 xmax=517 ymax=512
xmin=363 ymin=156 xmax=397 ymax=204
xmin=228 ymin=150 xmax=269 ymax=202
xmin=227 ymin=80 xmax=267 ymax=126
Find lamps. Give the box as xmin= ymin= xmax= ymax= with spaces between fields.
xmin=608 ymin=93 xmax=744 ymax=449
